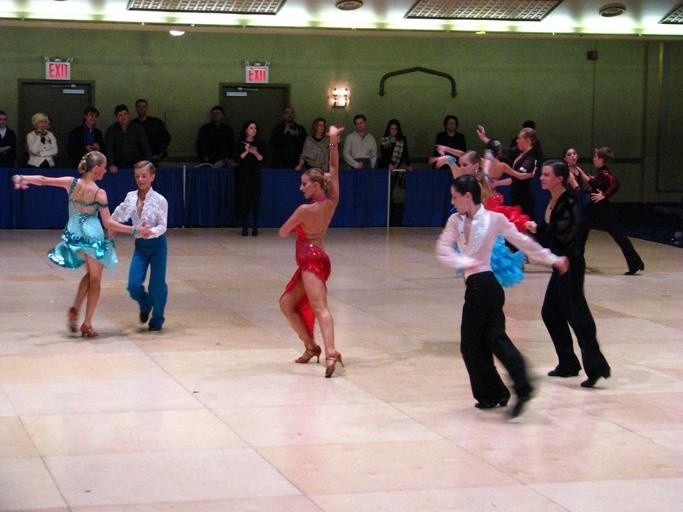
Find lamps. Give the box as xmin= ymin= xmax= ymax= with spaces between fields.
xmin=327 ymin=84 xmax=351 ymax=109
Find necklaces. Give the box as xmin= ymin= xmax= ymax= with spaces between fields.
xmin=549 ymin=191 xmax=561 ymax=210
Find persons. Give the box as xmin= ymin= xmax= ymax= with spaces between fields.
xmin=523 ymin=158 xmax=611 ymax=387
xmin=108 ymin=160 xmax=169 ymax=332
xmin=68 ymin=106 xmax=104 ymax=166
xmin=433 ymin=114 xmax=468 ymax=169
xmin=11 ymin=151 xmax=151 ymax=338
xmin=237 ymin=119 xmax=267 ymax=237
xmin=277 ymin=124 xmax=345 ymax=379
xmin=26 ymin=112 xmax=58 ymax=170
xmin=378 ymin=118 xmax=414 ymax=172
xmin=476 ymin=124 xmax=539 ymax=254
xmin=104 ymin=103 xmax=152 ymax=173
xmin=434 ymin=174 xmax=570 ymax=419
xmin=342 ymin=113 xmax=378 ymax=169
xmin=0 ymin=110 xmax=15 ymax=169
xmin=436 ymin=137 xmax=537 ymax=233
xmin=196 ymin=104 xmax=236 ymax=167
xmin=563 ymin=148 xmax=591 ymax=198
xmin=269 ymin=105 xmax=309 ymax=171
xmin=293 ymin=117 xmax=331 ymax=172
xmin=429 ymin=150 xmax=537 ymax=288
xmin=131 ymin=98 xmax=172 ymax=166
xmin=584 ymin=146 xmax=645 ymax=276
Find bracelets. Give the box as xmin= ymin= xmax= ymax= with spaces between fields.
xmin=131 ymin=225 xmax=136 ymax=235
xmin=329 ymin=142 xmax=338 ymax=147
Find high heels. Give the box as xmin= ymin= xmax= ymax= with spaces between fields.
xmin=296 ymin=346 xmax=321 ymax=363
xmin=66 ymin=307 xmax=78 ymax=334
xmin=325 ymin=352 xmax=343 ymax=377
xmin=80 ymin=324 xmax=98 ymax=340
xmin=474 ymin=396 xmax=512 ymax=409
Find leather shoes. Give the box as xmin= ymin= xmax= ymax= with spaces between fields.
xmin=624 ymin=263 xmax=646 ymax=275
xmin=581 ymin=366 xmax=610 ymax=387
xmin=548 ymin=365 xmax=581 ymax=378
xmin=149 ymin=325 xmax=164 ymax=331
xmin=140 ymin=301 xmax=151 ymax=324
